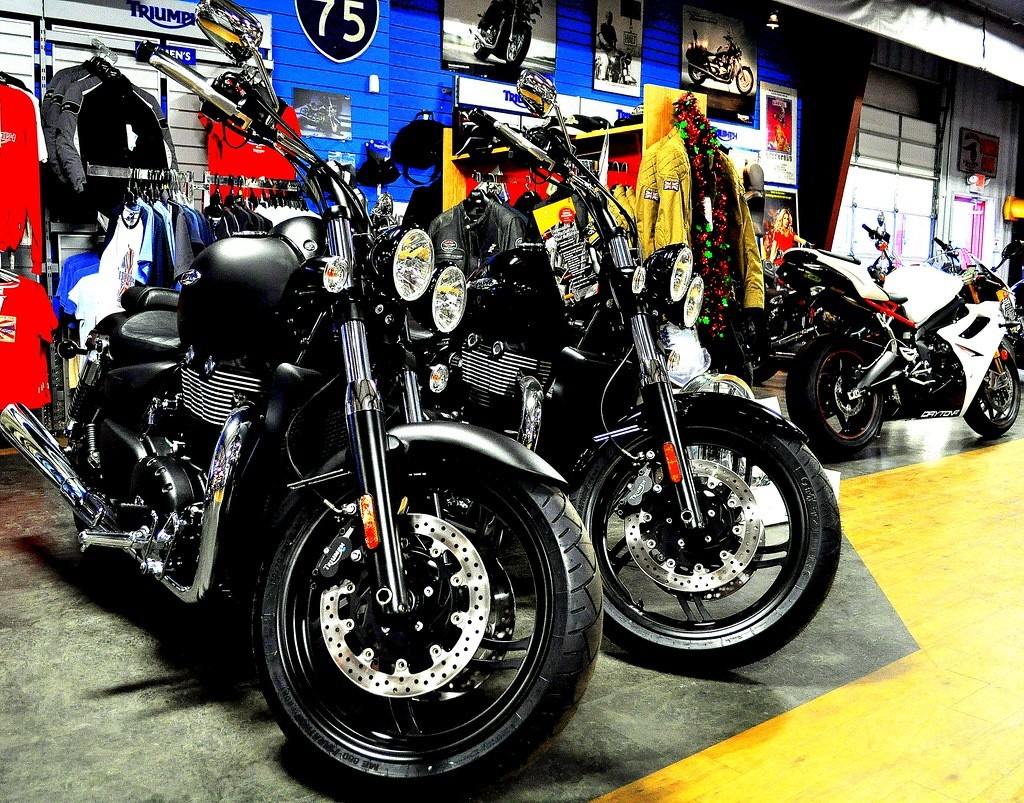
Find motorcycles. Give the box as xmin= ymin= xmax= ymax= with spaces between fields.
xmin=730 ymin=212 xmax=1023 ymax=461
xmin=471 ymin=0 xmax=539 ymax=64
xmin=292 ymin=96 xmax=342 ymax=132
xmin=252 ymin=73 xmax=845 ymax=677
xmin=0 ymin=1 xmax=603 ymax=803
xmin=772 ymin=111 xmax=787 ymax=129
xmin=595 ymin=40 xmax=633 ymax=87
xmin=686 ymin=26 xmax=753 ymax=95
